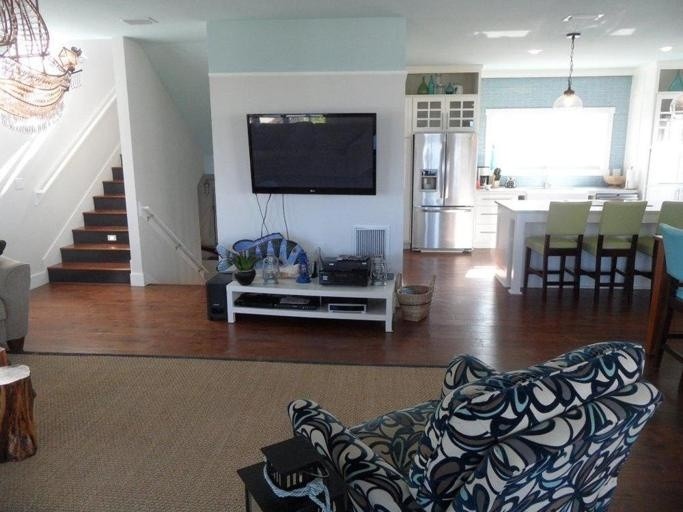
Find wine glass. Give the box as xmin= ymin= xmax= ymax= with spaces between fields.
xmin=602 ymin=175 xmax=626 ymax=190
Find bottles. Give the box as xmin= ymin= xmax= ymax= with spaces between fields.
xmin=415 ymin=75 xmax=434 ymax=94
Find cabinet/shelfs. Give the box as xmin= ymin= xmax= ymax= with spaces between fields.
xmin=477 ymin=194 xmax=513 ymax=243
xmin=406 ymin=66 xmax=482 ymax=135
xmin=225 ymin=276 xmax=396 ymax=334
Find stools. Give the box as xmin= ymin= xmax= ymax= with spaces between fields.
xmin=0 ymin=364 xmax=38 ymax=464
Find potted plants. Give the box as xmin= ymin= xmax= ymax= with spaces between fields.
xmin=492 ymin=167 xmax=502 ymax=188
xmin=227 ymin=249 xmax=260 ymax=286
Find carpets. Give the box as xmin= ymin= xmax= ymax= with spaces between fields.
xmin=0 ymin=350 xmax=449 ymax=512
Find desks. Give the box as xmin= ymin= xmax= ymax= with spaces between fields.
xmin=493 ymin=199 xmax=659 ymax=296
xmin=236 ymin=460 xmax=345 ymax=512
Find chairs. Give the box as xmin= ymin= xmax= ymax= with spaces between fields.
xmin=286 ymin=337 xmax=664 ymax=512
xmin=522 ymin=200 xmax=683 ymax=304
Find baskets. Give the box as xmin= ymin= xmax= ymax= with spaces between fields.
xmin=395 ymin=272 xmax=436 ymax=322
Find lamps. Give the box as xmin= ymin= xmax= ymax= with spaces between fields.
xmin=551 ymin=33 xmax=584 ymax=117
xmin=0 ymin=1 xmax=86 ymax=135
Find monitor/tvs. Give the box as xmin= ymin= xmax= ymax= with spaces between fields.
xmin=246 ymin=111 xmax=378 ymax=196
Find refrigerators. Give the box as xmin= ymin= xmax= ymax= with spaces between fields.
xmin=410 ymin=131 xmax=477 ymax=254
